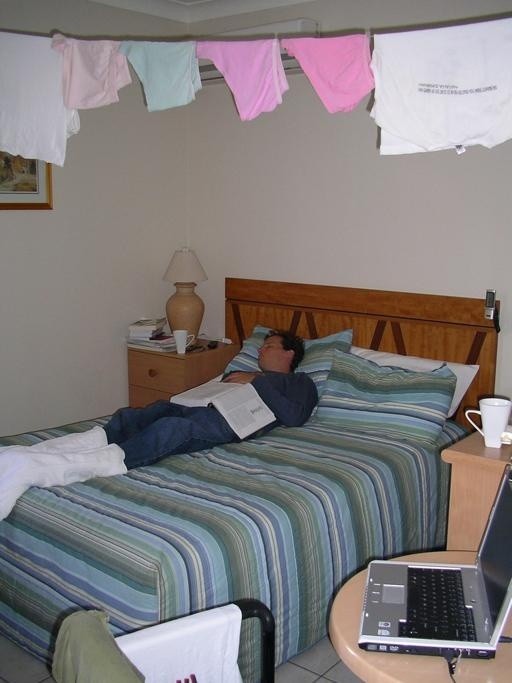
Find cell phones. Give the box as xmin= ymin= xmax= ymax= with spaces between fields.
xmin=484 ymin=289 xmax=496 ymax=319
xmin=207 ymin=341 xmax=217 ymax=349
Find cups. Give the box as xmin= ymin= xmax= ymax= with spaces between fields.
xmin=465 ymin=398 xmax=512 ymax=448
xmin=173 ymin=330 xmax=195 ymax=354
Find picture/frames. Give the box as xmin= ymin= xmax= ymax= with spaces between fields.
xmin=0 ymin=150 xmax=53 ymax=210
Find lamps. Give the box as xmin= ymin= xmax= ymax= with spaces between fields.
xmin=162 ymin=247 xmax=208 ymax=340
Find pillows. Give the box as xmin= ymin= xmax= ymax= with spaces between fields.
xmin=349 ymin=343 xmax=480 ymax=417
xmin=224 ymin=324 xmax=353 ymax=401
xmin=316 ymin=348 xmax=457 ymax=444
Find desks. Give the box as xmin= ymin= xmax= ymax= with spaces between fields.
xmin=328 ymin=550 xmax=512 ymax=683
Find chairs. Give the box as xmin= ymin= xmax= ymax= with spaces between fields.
xmin=70 ymin=599 xmax=280 ymax=678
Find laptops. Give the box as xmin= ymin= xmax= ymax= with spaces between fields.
xmin=358 ymin=463 xmax=512 ymax=659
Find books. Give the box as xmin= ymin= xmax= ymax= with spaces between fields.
xmin=170 ymin=380 xmax=277 ymax=440
xmin=127 ymin=317 xmax=176 ymax=353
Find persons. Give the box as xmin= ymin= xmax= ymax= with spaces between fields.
xmin=1 ymin=329 xmax=319 ymax=488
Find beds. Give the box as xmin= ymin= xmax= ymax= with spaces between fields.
xmin=0 ymin=278 xmax=500 ymax=683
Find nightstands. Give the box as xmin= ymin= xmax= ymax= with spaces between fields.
xmin=126 ymin=337 xmax=240 ymax=408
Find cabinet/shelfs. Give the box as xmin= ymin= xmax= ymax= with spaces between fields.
xmin=440 ymin=428 xmax=512 ymax=550
